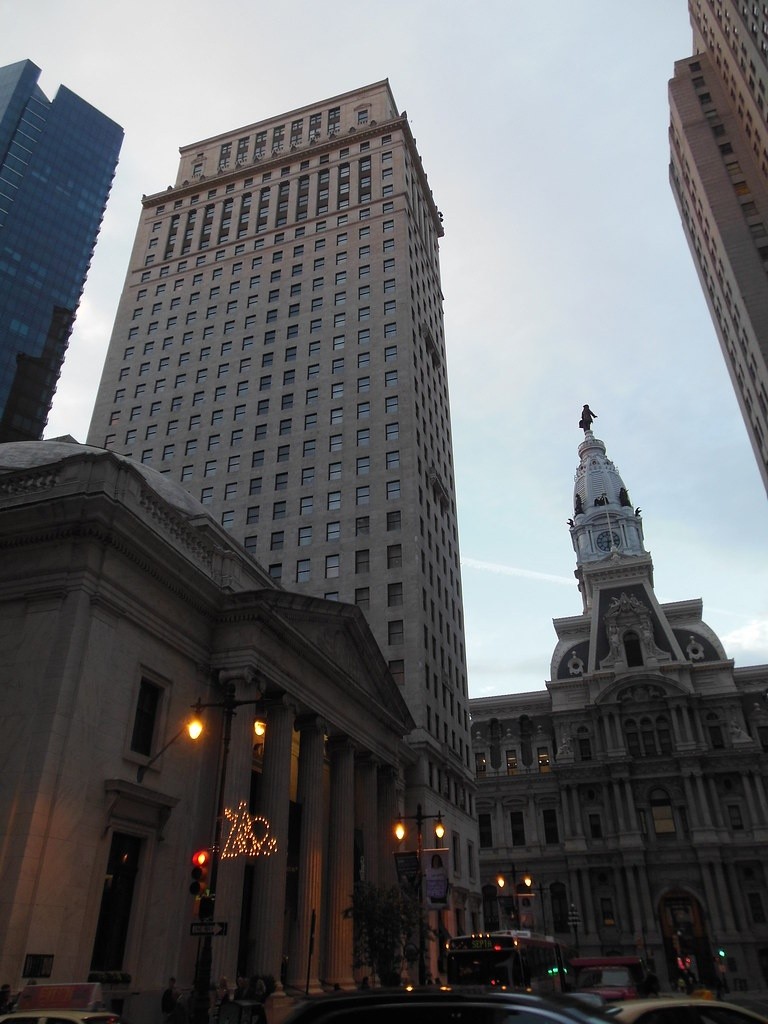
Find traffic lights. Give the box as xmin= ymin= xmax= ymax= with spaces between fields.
xmin=189 ymin=847 xmax=208 ymax=897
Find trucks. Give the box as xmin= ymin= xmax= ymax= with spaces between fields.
xmin=570 ymin=956 xmax=648 ymax=1000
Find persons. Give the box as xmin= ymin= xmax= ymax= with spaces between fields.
xmin=359 ymin=975 xmax=373 ymax=990
xmin=331 ymin=982 xmax=343 ymax=990
xmin=426 ymin=977 xmax=442 ymax=985
xmin=425 ymin=855 xmax=450 ymax=904
xmin=162 ymin=976 xmax=250 ymax=1023
xmin=644 ymin=968 xmax=662 ymax=997
xmin=0 ymin=984 xmax=16 ymax=1015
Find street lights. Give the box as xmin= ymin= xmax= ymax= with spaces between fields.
xmin=393 ymin=802 xmax=446 ymax=986
xmin=187 ymin=683 xmax=272 ymax=1024
xmin=496 ymin=862 xmax=532 ymax=931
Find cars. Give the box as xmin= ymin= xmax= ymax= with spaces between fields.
xmin=597 ymin=997 xmax=768 ymax=1024
xmin=563 ymin=992 xmax=609 ymax=1008
xmin=0 ymin=982 xmax=120 ymax=1024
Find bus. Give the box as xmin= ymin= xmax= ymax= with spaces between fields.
xmin=446 ymin=930 xmax=580 ymax=992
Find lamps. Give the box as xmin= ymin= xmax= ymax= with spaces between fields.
xmin=137 ymin=714 xmax=201 ymax=783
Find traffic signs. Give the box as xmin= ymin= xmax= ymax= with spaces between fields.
xmin=189 ymin=922 xmax=227 ymax=935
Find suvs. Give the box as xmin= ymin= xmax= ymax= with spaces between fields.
xmin=275 ymin=989 xmax=627 ymax=1024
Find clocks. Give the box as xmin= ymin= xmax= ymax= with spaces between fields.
xmin=597 ymin=531 xmax=620 ymax=552
